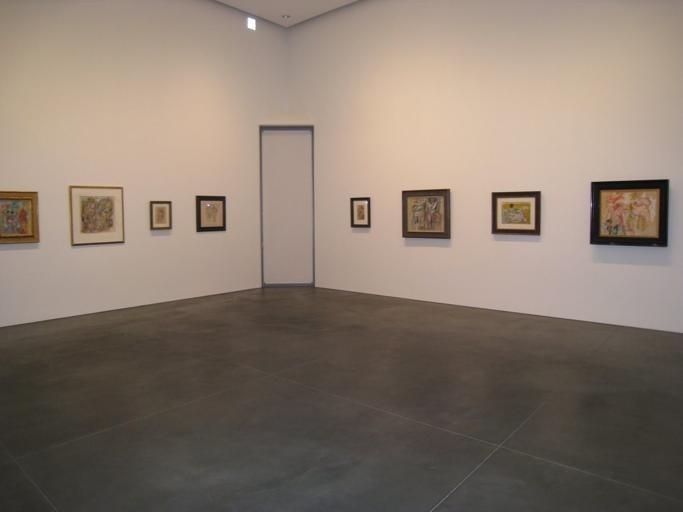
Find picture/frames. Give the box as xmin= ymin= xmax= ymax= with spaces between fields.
xmin=400 ymin=187 xmax=453 ymax=240
xmin=589 ymin=179 xmax=668 ymax=247
xmin=0 ymin=186 xmax=225 ymax=247
xmin=349 ymin=195 xmax=373 ymax=230
xmin=489 ymin=190 xmax=543 ymax=237
